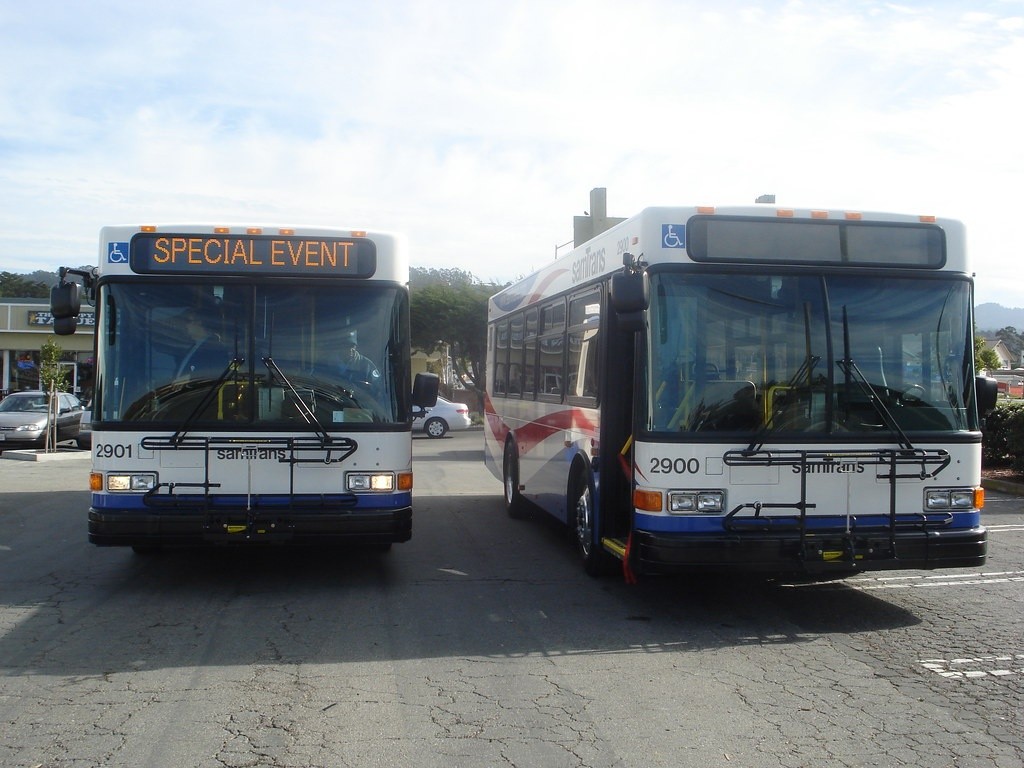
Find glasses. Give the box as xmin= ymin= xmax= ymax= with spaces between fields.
xmin=186 ymin=318 xmax=201 ymax=324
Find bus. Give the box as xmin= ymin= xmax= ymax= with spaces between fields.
xmin=479 ymin=202 xmax=991 ymax=602
xmin=50 ymin=223 xmax=443 ymax=572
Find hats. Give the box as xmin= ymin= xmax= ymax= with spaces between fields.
xmin=337 ymin=329 xmax=358 ymax=348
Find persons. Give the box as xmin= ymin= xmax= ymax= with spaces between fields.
xmin=172 ymin=309 xmax=228 ymax=384
xmin=314 ymin=330 xmax=381 ymax=396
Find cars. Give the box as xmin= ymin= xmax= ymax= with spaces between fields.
xmin=0 ymin=389 xmax=83 ymax=457
xmin=77 ymin=378 xmax=121 ymax=451
xmin=409 ymin=392 xmax=473 ymax=439
